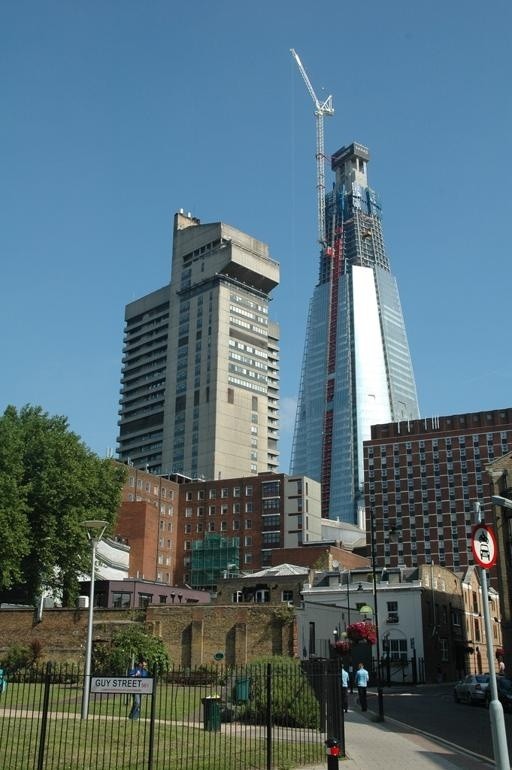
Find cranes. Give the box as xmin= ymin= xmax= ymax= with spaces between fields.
xmin=289 ymin=48 xmax=334 ymax=247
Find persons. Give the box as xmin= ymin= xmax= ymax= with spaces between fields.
xmin=354 ymin=663 xmax=370 ymax=712
xmin=128 ymin=658 xmax=149 ymax=720
xmin=0 ymin=662 xmax=4 ymax=696
xmin=499 ymin=660 xmax=505 ymax=672
xmin=341 ymin=664 xmax=349 ymax=713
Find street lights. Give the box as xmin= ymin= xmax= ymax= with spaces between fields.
xmin=333 ymin=630 xmax=338 ymax=647
xmin=39 ymin=586 xmax=53 ymax=621
xmin=80 ymin=520 xmax=109 ymax=720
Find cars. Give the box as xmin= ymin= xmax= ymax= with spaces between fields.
xmin=455 ymin=674 xmax=512 ymax=707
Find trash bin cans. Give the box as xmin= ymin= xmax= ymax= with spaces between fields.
xmin=200 ymin=697 xmax=221 ymax=732
xmin=235 ymin=679 xmax=250 ymax=706
